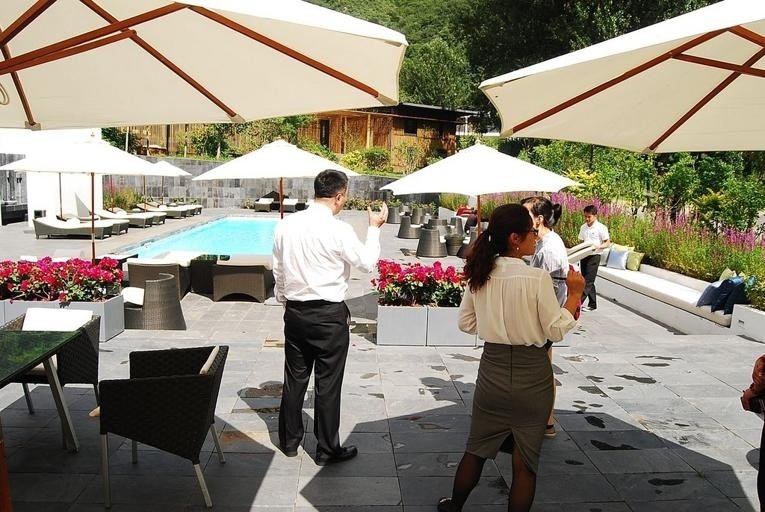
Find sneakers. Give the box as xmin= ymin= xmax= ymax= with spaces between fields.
xmin=544 ymin=423 xmax=557 ymax=437
xmin=581 ymin=306 xmax=598 ymax=313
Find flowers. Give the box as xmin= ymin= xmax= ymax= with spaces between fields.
xmin=369 ymin=256 xmax=426 ymax=308
xmin=0 ymin=255 xmax=123 ymax=303
xmin=424 ymin=260 xmax=469 ymax=307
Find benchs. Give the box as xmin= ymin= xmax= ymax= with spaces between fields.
xmin=569 ymin=242 xmax=740 ymax=335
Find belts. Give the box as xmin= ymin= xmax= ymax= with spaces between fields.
xmin=285 ymin=299 xmax=333 ymax=307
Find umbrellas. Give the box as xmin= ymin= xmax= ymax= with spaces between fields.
xmin=0 ymin=134 xmax=193 ymax=265
xmin=380 ymin=139 xmax=584 ymax=237
xmin=478 ymin=0 xmax=765 ymax=157
xmin=0 ymin=0 xmax=408 ymax=132
xmin=193 ymin=137 xmax=360 ymax=218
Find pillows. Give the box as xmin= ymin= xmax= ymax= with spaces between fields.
xmin=596 ymin=240 xmax=647 ymax=272
xmin=696 ymin=266 xmax=753 ymax=317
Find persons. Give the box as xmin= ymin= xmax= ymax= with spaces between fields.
xmin=519 ymin=196 xmax=570 ymax=439
xmin=437 ymin=203 xmax=587 ymax=512
xmin=578 ymin=205 xmax=610 ymax=312
xmin=456 ymin=203 xmax=479 ymax=235
xmin=741 ymin=355 xmax=765 ymax=512
xmin=271 ymin=169 xmax=389 ymax=466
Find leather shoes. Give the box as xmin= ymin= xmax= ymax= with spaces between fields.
xmin=314 ymin=445 xmax=358 ymax=467
xmin=284 ymin=448 xmax=297 ymax=457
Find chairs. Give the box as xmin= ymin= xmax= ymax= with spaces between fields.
xmin=386 ymin=204 xmax=481 ymax=258
xmin=97 ymin=342 xmax=232 ymax=510
xmin=280 ymin=197 xmax=300 ymax=213
xmin=0 ymin=307 xmax=103 ymax=414
xmin=211 ymin=253 xmax=275 ymax=304
xmin=127 ymin=248 xmax=208 ymax=300
xmin=21 ymin=247 xmax=87 ymax=263
xmin=123 ymin=269 xmax=185 ymax=329
xmin=253 ymin=196 xmax=275 ymax=212
xmin=32 ymin=198 xmax=203 ymax=241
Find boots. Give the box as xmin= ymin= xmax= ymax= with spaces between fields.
xmin=437 ymin=496 xmax=453 ymax=512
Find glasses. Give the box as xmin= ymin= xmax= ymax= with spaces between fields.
xmin=528 ymin=228 xmax=539 ymax=237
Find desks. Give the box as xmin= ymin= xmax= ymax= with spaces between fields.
xmin=188 ymin=254 xmax=231 ymax=294
xmin=0 ymin=328 xmax=84 ymax=452
xmin=271 ymin=202 xmax=280 ymax=210
xmin=295 ymin=203 xmax=305 ymax=210
xmin=91 ymin=251 xmax=137 ymax=273
